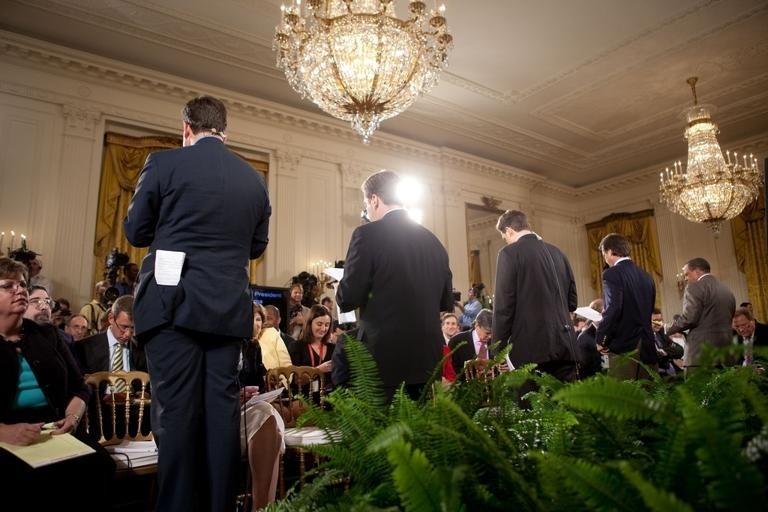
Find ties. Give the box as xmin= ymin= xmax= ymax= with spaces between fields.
xmin=112 ymin=343 xmax=132 ymax=395
xmin=478 ymin=341 xmax=487 ymax=374
xmin=745 ymin=337 xmax=752 ymax=366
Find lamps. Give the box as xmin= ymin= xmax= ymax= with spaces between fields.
xmin=272 ymin=0 xmax=456 ymax=141
xmin=657 ymin=77 xmax=764 ymax=239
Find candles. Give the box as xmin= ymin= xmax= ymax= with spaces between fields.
xmin=312 ymin=260 xmax=333 ymax=277
xmin=0 ymin=230 xmax=26 ymax=258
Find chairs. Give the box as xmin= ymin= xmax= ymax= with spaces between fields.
xmin=82 ymin=371 xmax=159 ymax=512
xmin=464 ymin=358 xmax=502 ymax=407
xmin=267 ymin=365 xmax=352 ymax=505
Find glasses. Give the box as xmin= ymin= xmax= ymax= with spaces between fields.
xmin=114 ymin=319 xmax=134 ymax=332
xmin=31 ymin=298 xmax=56 ymax=308
xmin=0 ymin=282 xmax=33 ymax=292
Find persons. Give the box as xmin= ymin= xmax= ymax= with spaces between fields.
xmin=490 ymin=207 xmax=580 ymax=402
xmin=124 ymin=95 xmax=274 ymax=512
xmin=594 ymin=232 xmax=664 ymax=389
xmin=1 ymin=257 xmax=768 ymax=510
xmin=656 ymin=257 xmax=734 ymax=385
xmin=332 ymin=167 xmax=454 ymax=415
